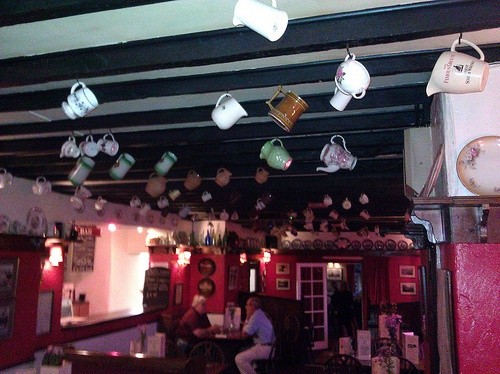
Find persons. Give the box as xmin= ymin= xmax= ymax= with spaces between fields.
xmin=176 ymin=294 xmax=222 ymax=363
xmin=330 ymin=283 xmax=340 ymax=307
xmin=336 ymin=282 xmax=355 ymax=347
xmin=234 ymin=297 xmax=276 ymax=374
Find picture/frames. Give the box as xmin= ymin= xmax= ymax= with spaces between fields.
xmin=401 ymin=282 xmax=418 ymax=296
xmin=399 ymin=264 xmax=416 ymax=280
xmin=326 ymin=268 xmax=344 ymax=281
xmin=0 ymin=255 xmax=22 ymax=340
xmin=275 ymin=262 xmax=291 ymax=275
xmin=276 ymin=277 xmax=290 ymax=291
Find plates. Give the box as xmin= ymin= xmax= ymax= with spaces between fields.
xmin=455 ymin=136 xmax=500 ymax=196
xmin=25 ymin=205 xmax=47 ymax=236
xmin=279 ymin=237 xmax=410 ymax=251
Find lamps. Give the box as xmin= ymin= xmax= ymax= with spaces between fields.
xmin=50 ymin=246 xmax=63 ymax=267
xmin=176 ymin=250 xmax=191 ymax=268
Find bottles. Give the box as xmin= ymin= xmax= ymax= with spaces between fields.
xmin=149 ymin=226 xmax=272 ymax=251
xmin=69 ymin=219 xmax=79 ymax=240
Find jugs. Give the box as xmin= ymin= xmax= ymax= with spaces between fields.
xmin=260 ymin=139 xmax=293 ymax=171
xmin=315 ymin=135 xmax=357 ymax=173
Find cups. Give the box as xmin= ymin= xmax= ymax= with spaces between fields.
xmin=334 ymin=54 xmax=371 ymax=100
xmin=265 ymin=86 xmax=309 ymax=133
xmin=63 ymin=82 xmax=98 ymax=120
xmin=0 ymin=133 xmax=267 ymax=223
xmin=425 ymin=39 xmax=489 ymax=98
xmin=210 ymin=93 xmax=248 ymax=130
xmin=283 ymin=193 xmax=372 ymax=239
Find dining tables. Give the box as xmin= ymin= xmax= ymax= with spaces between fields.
xmin=197 ymin=329 xmax=255 ymax=374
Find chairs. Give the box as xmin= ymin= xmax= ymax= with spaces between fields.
xmin=160 ymin=314 xmax=177 ymax=339
xmin=323 ymin=353 xmax=363 ymax=374
xmin=256 ymin=308 xmax=303 ymax=372
